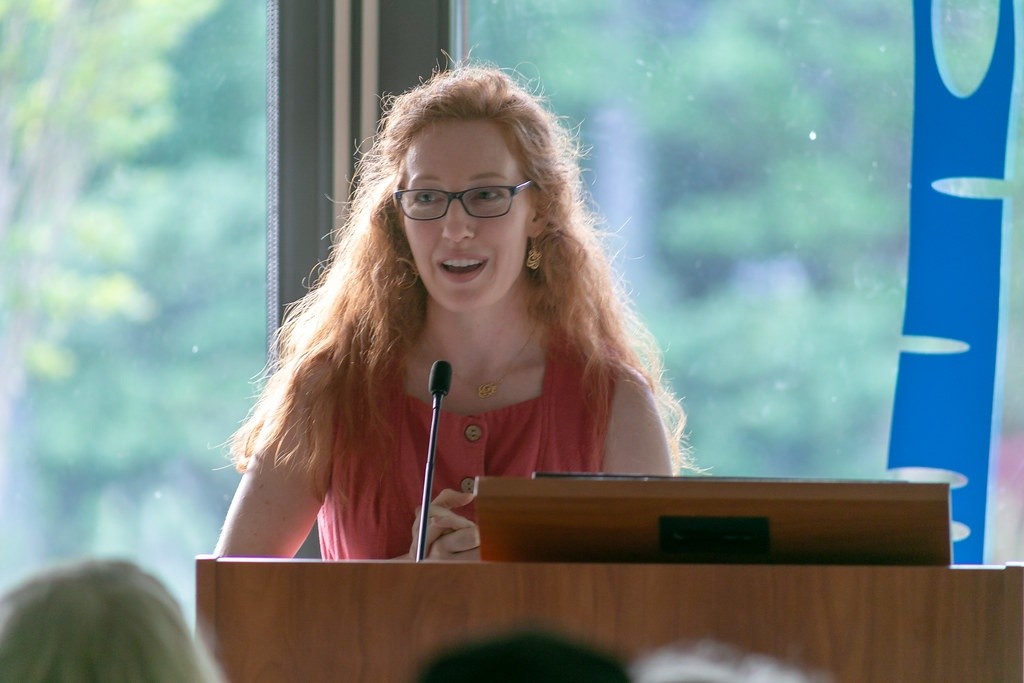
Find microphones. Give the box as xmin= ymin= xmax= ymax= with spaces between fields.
xmin=414 ymin=359 xmax=452 ymax=561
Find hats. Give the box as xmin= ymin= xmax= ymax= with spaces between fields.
xmin=0 ymin=558 xmax=210 ymax=683
xmin=418 ymin=630 xmax=632 ymax=683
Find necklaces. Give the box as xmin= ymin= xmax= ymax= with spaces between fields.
xmin=419 ymin=319 xmax=537 ymax=399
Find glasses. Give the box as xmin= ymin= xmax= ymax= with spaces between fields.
xmin=394 ymin=180 xmax=534 ymax=220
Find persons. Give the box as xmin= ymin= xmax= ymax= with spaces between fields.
xmin=213 ymin=63 xmax=688 ymax=561
xmin=1 ymin=551 xmax=807 ymax=683
